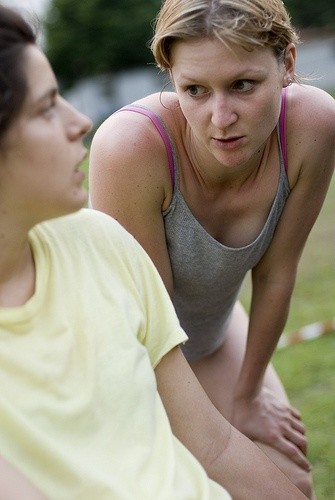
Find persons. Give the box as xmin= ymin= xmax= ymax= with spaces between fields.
xmin=89 ymin=1 xmax=334 ymax=500
xmin=1 ymin=7 xmax=312 ymax=500
